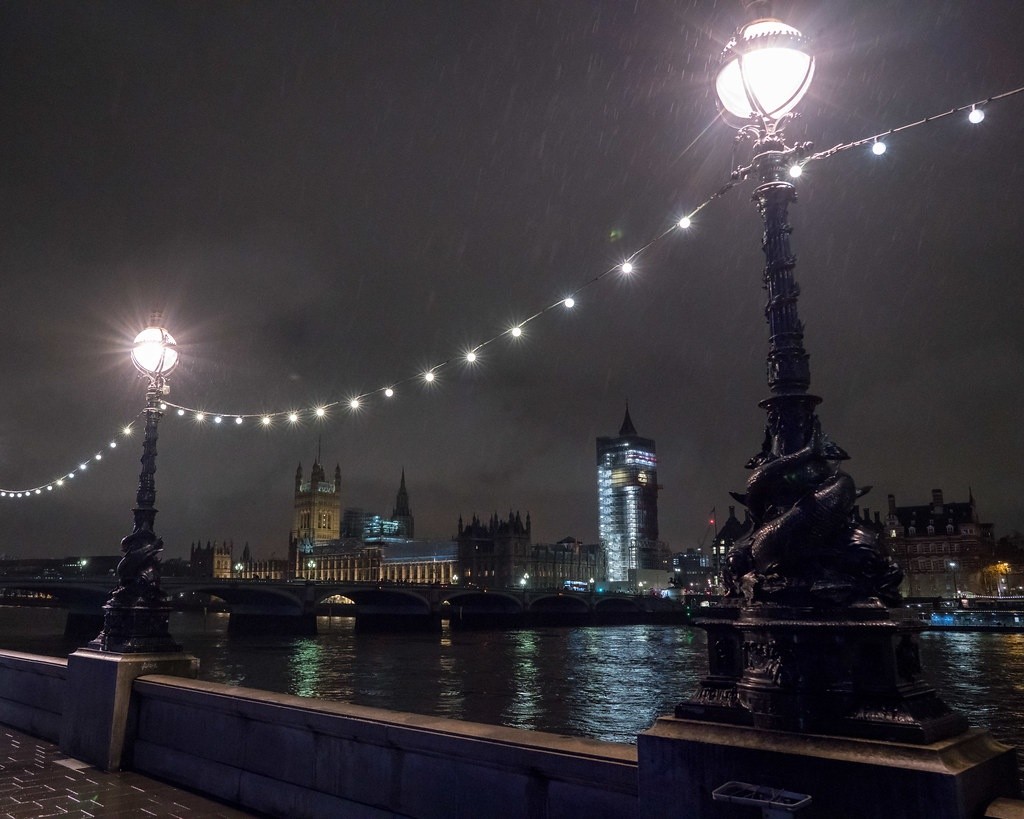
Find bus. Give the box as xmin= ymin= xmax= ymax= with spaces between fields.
xmin=563 ymin=580 xmax=588 ymax=592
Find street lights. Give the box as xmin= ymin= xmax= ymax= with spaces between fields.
xmin=90 ymin=326 xmax=180 ymax=665
xmin=669 ymin=16 xmax=965 ymax=742
xmin=949 ymin=555 xmax=958 ymax=598
